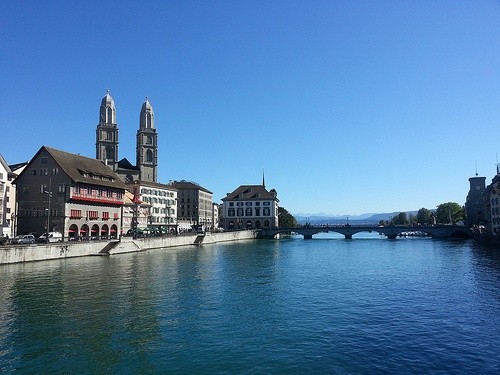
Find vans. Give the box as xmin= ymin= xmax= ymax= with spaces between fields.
xmin=41 ymin=232 xmax=61 ymax=244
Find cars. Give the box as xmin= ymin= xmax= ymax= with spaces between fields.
xmin=12 ymin=235 xmax=35 ymax=245
